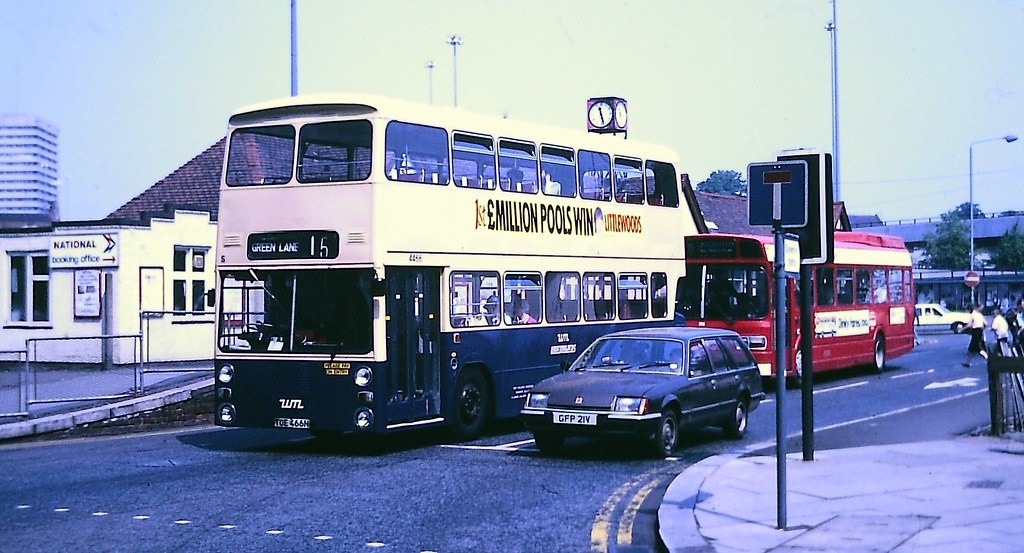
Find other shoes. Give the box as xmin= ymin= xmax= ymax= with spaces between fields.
xmin=960 ymin=362 xmax=971 ymax=367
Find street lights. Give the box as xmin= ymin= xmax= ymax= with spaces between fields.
xmin=967 ymin=134 xmax=1019 ymax=299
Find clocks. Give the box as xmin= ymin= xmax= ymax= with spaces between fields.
xmin=586 ymin=97 xmax=628 ymax=140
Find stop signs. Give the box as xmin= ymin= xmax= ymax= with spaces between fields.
xmin=964 ymin=270 xmax=980 ymax=288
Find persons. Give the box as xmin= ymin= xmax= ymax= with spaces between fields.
xmin=670 ymin=347 xmax=683 ymax=367
xmin=956 ymin=299 xmax=988 ymax=367
xmin=706 ymin=269 xmax=764 ymax=319
xmin=483 ymin=295 xmax=537 ymax=324
xmin=602 ymin=341 xmax=643 ymax=364
xmin=989 ymin=308 xmax=1012 ymax=357
xmin=1006 ymin=299 xmax=1024 ymax=357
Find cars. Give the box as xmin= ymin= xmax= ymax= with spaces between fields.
xmin=519 ymin=323 xmax=765 ymax=456
xmin=910 ymin=304 xmax=975 ymax=333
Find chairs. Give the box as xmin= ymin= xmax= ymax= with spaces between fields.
xmin=397 ymin=168 xmax=665 ymax=206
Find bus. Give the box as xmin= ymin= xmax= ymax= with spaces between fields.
xmin=204 ymin=89 xmax=713 ymax=440
xmin=683 ymin=225 xmax=918 ymax=385
